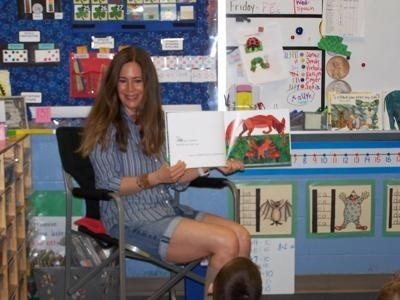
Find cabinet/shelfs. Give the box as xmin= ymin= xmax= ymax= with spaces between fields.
xmin=0 ymin=132 xmax=35 ymax=300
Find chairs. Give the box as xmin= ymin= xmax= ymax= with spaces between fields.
xmin=56 ymin=125 xmax=239 ymax=300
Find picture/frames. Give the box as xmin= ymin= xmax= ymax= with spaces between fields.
xmin=235 ymin=181 xmax=297 ymax=238
xmin=383 ymin=179 xmax=400 ymax=235
xmin=305 ymin=180 xmax=374 ymax=237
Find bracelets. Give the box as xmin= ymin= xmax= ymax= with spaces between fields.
xmin=198 ymin=168 xmax=210 ymax=177
xmin=136 ymin=172 xmax=152 ymax=190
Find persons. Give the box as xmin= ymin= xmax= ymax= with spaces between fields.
xmin=73 ymin=44 xmax=252 ymax=300
